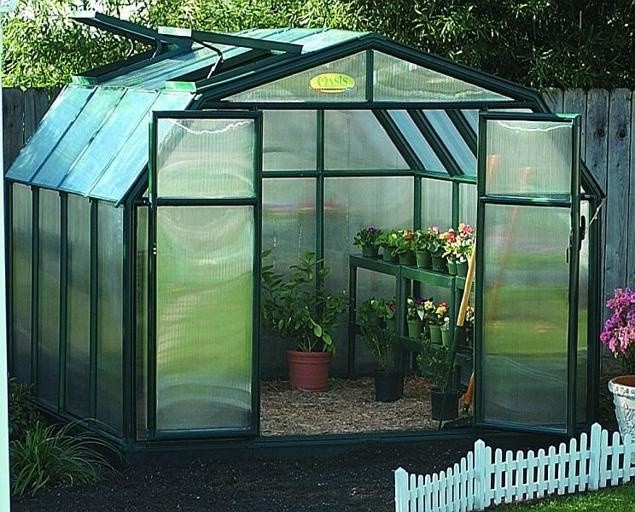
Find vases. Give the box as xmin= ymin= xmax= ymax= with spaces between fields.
xmin=607 ymin=371 xmax=634 ymax=470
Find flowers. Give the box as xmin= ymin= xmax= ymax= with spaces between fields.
xmin=352 ymin=222 xmax=477 ymax=346
xmin=598 ymin=283 xmax=633 ymax=382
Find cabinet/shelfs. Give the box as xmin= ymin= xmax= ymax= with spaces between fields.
xmin=343 ymin=252 xmax=477 ymax=378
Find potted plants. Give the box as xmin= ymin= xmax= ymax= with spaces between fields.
xmin=413 ymin=335 xmax=461 ymax=423
xmin=355 ymin=308 xmax=408 ymax=402
xmin=260 ymin=246 xmax=353 ymax=392
